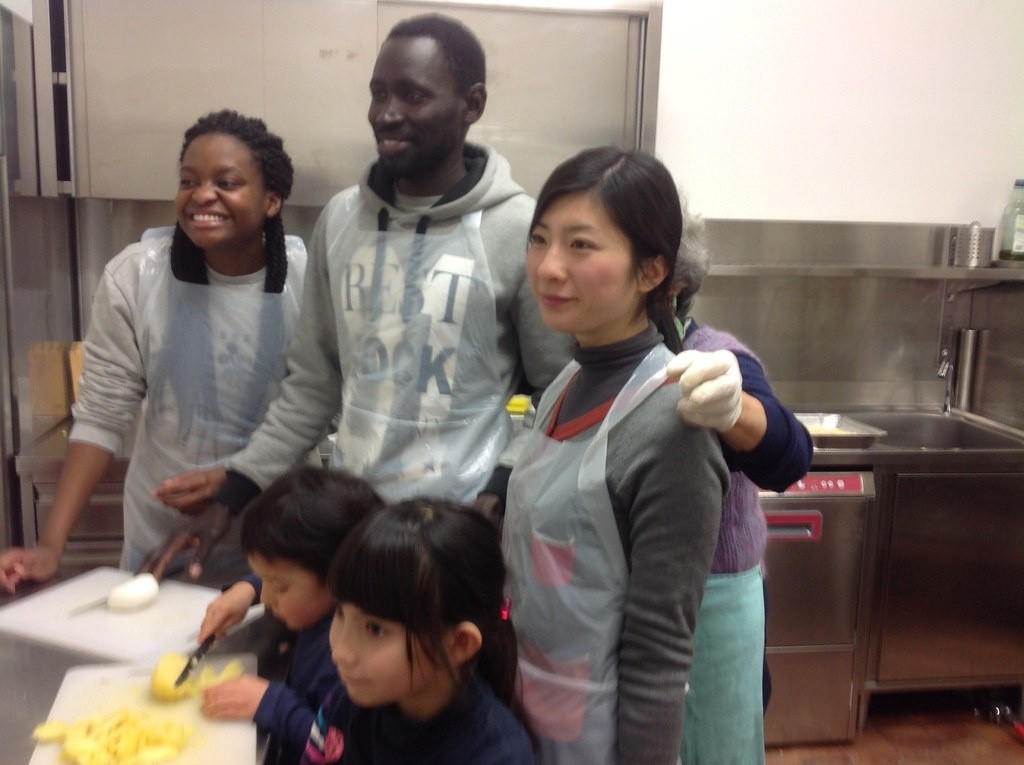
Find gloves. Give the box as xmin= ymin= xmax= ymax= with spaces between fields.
xmin=666 ymin=350 xmax=743 ymax=433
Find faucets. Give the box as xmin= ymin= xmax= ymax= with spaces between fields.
xmin=937 ymin=357 xmax=955 ymax=416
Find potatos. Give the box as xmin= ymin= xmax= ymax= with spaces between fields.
xmin=32 ymin=655 xmax=247 ymax=765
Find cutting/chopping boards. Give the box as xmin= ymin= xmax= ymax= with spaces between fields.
xmin=28 ymin=649 xmax=263 ymax=764
xmin=0 ymin=566 xmax=231 ymax=669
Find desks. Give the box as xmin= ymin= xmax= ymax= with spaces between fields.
xmin=0 ymin=580 xmax=299 ymax=765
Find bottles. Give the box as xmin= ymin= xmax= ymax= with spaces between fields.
xmin=998 ymin=177 xmax=1024 ymax=262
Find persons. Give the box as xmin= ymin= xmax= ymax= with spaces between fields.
xmin=500 ymin=144 xmax=730 ymax=765
xmin=663 ymin=191 xmax=814 ymax=765
xmin=195 ymin=466 xmax=388 ymax=750
xmin=0 ymin=110 xmax=323 ymax=594
xmin=135 ymin=11 xmax=576 ymax=585
xmin=300 ymin=496 xmax=534 ymax=765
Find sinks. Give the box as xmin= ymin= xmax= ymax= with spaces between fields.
xmin=844 ymin=405 xmax=1024 ymax=466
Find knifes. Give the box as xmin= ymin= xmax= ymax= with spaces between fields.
xmin=175 ymin=632 xmax=218 ymax=689
